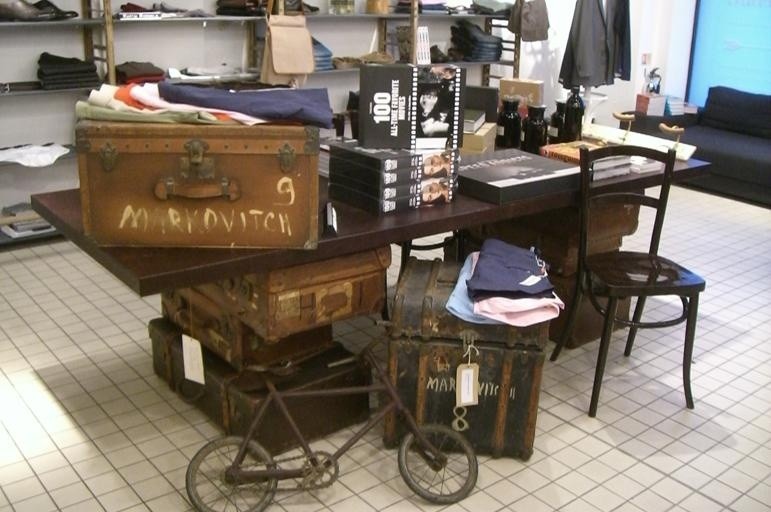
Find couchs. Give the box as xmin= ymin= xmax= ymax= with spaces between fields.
xmin=620 ymin=86 xmax=771 ymax=207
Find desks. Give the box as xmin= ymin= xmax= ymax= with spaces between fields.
xmin=30 ymin=134 xmax=712 ymax=298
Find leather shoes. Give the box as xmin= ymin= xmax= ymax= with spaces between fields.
xmin=0 ymin=1 xmax=58 ymax=22
xmin=33 ymin=0 xmax=78 ymax=20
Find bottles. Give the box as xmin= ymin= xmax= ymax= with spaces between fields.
xmin=494 ymin=96 xmax=521 ymax=151
xmin=549 ymin=84 xmax=585 ymax=145
xmin=520 ymin=104 xmax=547 ymax=156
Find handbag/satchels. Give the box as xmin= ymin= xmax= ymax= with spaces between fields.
xmin=215 ymin=0 xmax=306 ymax=16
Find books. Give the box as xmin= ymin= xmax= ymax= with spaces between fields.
xmin=540 ymin=139 xmax=631 ymax=169
xmin=464 ymin=108 xmax=486 ymax=135
xmin=500 ymin=77 xmax=547 ymax=123
xmin=636 ymin=93 xmax=699 ymax=119
xmin=319 ymin=135 xmax=463 ymax=216
xmin=592 ymin=164 xmax=631 ymax=181
xmin=259 ymin=16 xmax=316 ymax=89
xmin=632 ymin=156 xmax=663 ymax=173
xmin=356 ymin=62 xmax=468 ymax=151
xmin=1 ymin=203 xmax=57 ymax=242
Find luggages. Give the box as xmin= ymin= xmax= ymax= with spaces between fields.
xmin=443 ymin=189 xmax=649 ymax=351
xmin=73 ymin=114 xmax=322 ymax=253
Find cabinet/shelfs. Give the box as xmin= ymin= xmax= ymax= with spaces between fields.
xmin=0 ymin=0 xmax=522 ymax=248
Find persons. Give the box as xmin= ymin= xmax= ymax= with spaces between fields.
xmin=417 ymin=93 xmax=455 ymax=124
xmin=416 ymin=117 xmax=455 ymax=138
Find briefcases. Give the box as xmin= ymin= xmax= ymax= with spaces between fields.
xmin=148 ymin=244 xmax=393 ymax=457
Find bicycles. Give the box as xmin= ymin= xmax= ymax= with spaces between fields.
xmin=187 ymin=317 xmax=480 ymax=512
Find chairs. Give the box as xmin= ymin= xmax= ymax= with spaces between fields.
xmin=333 ymin=108 xmax=465 ymax=282
xmin=549 ymin=145 xmax=705 ymax=418
